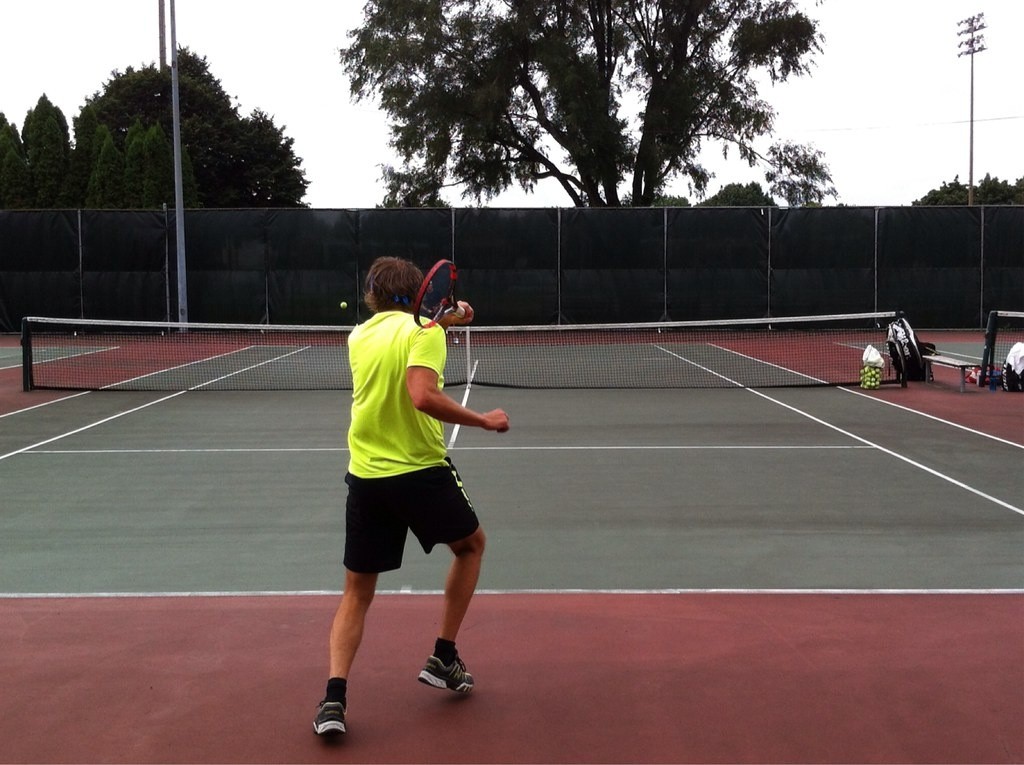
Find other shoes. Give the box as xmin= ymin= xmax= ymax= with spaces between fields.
xmin=454 ymin=338 xmax=459 ymax=344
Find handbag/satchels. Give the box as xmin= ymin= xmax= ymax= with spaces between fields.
xmin=966 ymin=365 xmax=1003 ymax=387
xmin=862 ymin=345 xmax=885 ymax=368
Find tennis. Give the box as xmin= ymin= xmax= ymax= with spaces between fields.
xmin=340 ymin=301 xmax=348 ymax=309
xmin=859 ymin=365 xmax=882 ymax=391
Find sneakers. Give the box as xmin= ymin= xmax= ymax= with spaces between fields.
xmin=312 ymin=701 xmax=346 ymax=734
xmin=418 ymin=655 xmax=474 ymax=692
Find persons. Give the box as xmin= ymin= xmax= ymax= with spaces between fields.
xmin=313 ymin=257 xmax=511 ymax=738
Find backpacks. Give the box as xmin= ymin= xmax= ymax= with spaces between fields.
xmin=886 ymin=317 xmax=936 ymax=382
xmin=1002 ymin=342 xmax=1024 ymax=392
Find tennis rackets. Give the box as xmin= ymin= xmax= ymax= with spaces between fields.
xmin=412 ymin=258 xmax=466 ymax=329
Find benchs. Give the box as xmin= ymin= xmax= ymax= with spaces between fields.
xmin=922 ymin=355 xmax=979 ymax=392
